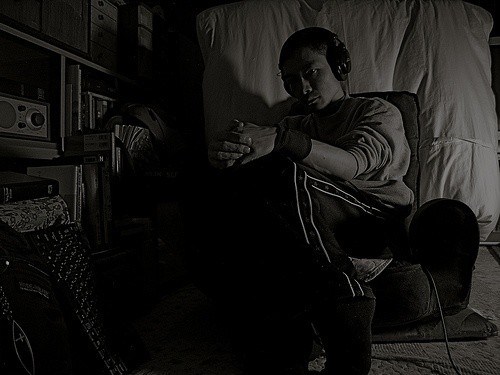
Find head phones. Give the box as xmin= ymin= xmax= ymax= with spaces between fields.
xmin=277 ymin=28 xmax=351 ymax=96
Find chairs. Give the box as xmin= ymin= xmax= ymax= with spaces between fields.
xmin=285 ymin=90 xmax=498 ymax=344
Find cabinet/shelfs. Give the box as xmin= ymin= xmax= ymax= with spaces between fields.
xmin=0 ymin=0 xmax=153 ymax=76
xmin=0 ymin=23 xmax=126 ymax=256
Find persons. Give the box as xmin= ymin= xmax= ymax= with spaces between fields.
xmin=208 ymin=26 xmax=418 ymax=375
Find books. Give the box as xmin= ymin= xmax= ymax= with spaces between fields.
xmin=24 ymin=165 xmax=86 ymax=229
xmin=66 ymin=62 xmax=114 ymax=135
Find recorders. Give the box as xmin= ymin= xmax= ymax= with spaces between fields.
xmin=0 ymin=76 xmax=51 ymax=142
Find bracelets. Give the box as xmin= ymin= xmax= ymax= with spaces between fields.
xmin=274 ymin=126 xmax=313 ymax=162
